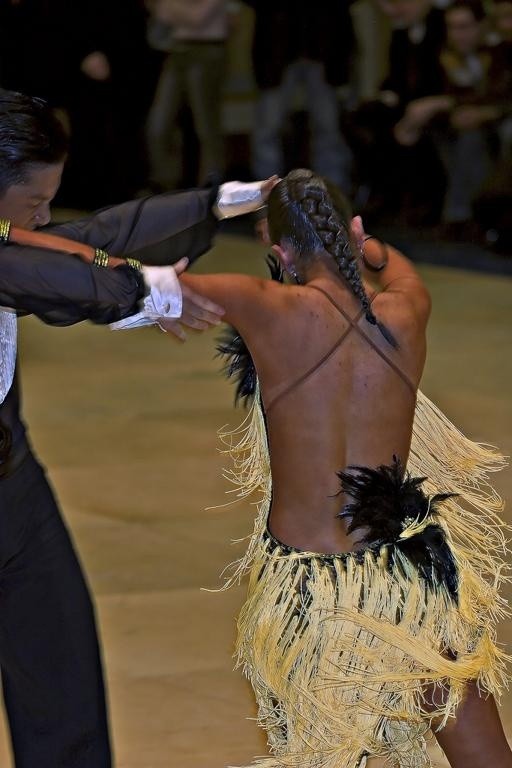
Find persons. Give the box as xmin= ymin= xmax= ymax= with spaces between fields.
xmin=1 ymin=164 xmax=512 ymax=767
xmin=1 ymin=88 xmax=285 ymax=767
xmin=346 ymin=2 xmax=509 ymax=263
xmin=242 ymin=2 xmax=362 ymax=191
xmin=143 ymin=2 xmax=243 ymax=199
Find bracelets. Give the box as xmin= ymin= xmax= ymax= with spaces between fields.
xmin=1 ymin=218 xmax=12 ymax=243
